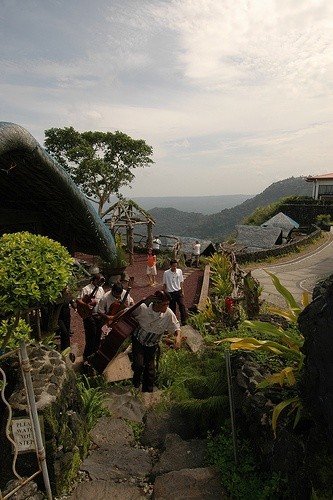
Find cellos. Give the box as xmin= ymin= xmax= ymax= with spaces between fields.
xmin=90 ymin=290 xmax=167 ymax=373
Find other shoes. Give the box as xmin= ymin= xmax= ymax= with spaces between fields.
xmin=150 ymin=283 xmax=155 ymax=287
xmin=148 ymin=279 xmax=152 ymax=284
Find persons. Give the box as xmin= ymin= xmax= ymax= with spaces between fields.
xmin=76 ymin=274 xmax=105 ymax=357
xmin=152 ymin=236 xmax=161 ymax=255
xmin=98 ymin=283 xmax=135 ymax=347
xmin=146 ymin=248 xmax=157 ymax=286
xmin=190 ymin=241 xmax=200 ymax=268
xmin=57 ymin=285 xmax=77 ymax=351
xmin=106 ymin=290 xmax=181 ymax=392
xmin=163 ymin=260 xmax=187 ymax=326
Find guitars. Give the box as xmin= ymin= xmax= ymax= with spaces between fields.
xmin=77 ymin=277 xmax=106 ymax=318
xmin=104 ymin=277 xmax=135 ymax=323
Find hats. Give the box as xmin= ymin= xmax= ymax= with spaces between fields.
xmin=155 ymin=289 xmax=171 ymax=302
